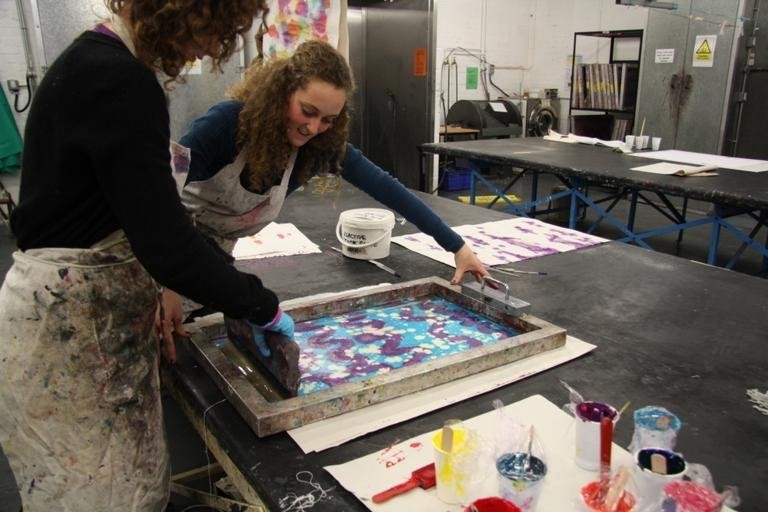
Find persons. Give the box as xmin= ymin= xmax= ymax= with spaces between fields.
xmin=151 ymin=40 xmax=503 ymax=370
xmin=0 ymin=2 xmax=297 ymax=508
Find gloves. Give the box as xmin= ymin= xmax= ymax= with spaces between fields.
xmin=245 ymin=307 xmax=295 ymax=357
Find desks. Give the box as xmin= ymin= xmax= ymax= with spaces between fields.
xmin=155 ymin=136 xmax=768 ymax=512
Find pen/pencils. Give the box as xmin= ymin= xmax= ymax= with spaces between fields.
xmin=640 ymin=115 xmax=646 ymax=137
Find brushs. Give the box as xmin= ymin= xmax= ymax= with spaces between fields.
xmin=224 ymin=310 xmax=300 ymax=403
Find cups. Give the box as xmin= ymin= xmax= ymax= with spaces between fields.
xmin=663 ymin=480 xmax=724 ymax=512
xmin=633 ymin=448 xmax=688 ymax=502
xmin=634 ymin=406 xmax=682 ymax=451
xmin=432 ymin=427 xmax=482 ymax=504
xmin=463 ymin=496 xmax=522 ymax=512
xmin=495 ymin=451 xmax=548 ymax=512
xmin=573 ymin=400 xmax=620 ymax=472
xmin=579 ymin=481 xmax=638 ymax=512
xmin=624 ymin=134 xmax=661 ymax=152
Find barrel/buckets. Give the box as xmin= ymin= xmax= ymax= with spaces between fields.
xmin=336 ymin=207 xmax=396 ymax=261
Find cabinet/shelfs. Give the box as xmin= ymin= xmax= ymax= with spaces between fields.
xmin=569 ymin=28 xmax=644 ymax=144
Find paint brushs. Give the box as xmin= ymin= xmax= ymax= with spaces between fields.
xmin=487 ymin=267 xmax=548 ymax=277
xmin=331 ymin=246 xmax=402 ymax=278
